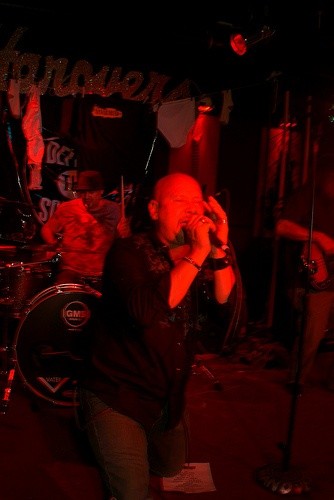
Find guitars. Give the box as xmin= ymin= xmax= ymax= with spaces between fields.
xmin=302 ymin=239 xmax=334 ymax=290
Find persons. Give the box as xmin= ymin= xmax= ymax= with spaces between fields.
xmin=40 ymin=171 xmax=132 ymax=294
xmin=274 ymin=165 xmax=334 ymax=396
xmin=81 ymin=174 xmax=248 ymax=500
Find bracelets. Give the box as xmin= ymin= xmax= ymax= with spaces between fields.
xmin=52 ymin=240 xmax=61 ymax=250
xmin=183 ymin=256 xmax=202 ymax=271
xmin=209 ymin=254 xmax=231 ymax=270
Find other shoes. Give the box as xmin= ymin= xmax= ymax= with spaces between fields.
xmin=285 ymin=383 xmax=303 ymax=394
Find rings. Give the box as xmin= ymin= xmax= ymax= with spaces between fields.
xmin=222 ymin=219 xmax=226 ymax=224
xmin=201 ymin=219 xmax=206 ymax=223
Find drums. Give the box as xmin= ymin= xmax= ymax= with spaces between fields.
xmin=12 ymin=285 xmax=104 ymax=408
xmin=0 ymin=246 xmax=61 ymax=315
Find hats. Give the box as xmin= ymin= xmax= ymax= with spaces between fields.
xmin=76 ymin=170 xmax=103 ymax=189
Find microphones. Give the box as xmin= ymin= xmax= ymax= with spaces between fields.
xmin=209 ymin=229 xmax=231 ymax=254
